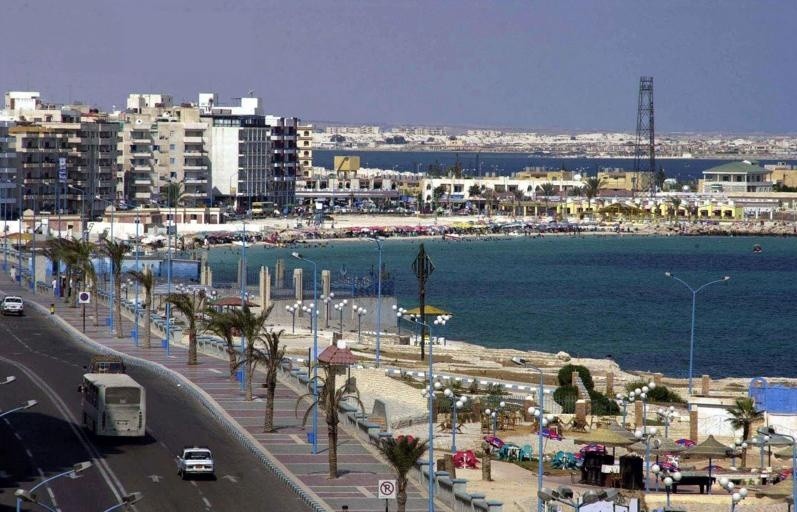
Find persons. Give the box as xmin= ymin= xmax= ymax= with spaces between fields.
xmin=9 ymin=265 xmax=17 ymax=282
xmin=50 ymin=277 xmax=57 ymax=296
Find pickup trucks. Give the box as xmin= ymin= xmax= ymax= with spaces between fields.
xmin=177 ymin=446 xmax=215 ymax=479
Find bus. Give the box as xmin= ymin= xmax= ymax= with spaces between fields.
xmin=76 ymin=372 xmax=148 ymax=444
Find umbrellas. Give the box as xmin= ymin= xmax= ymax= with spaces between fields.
xmin=650 ymin=460 xmax=681 ymax=472
xmin=773 ymin=442 xmax=796 ymax=459
xmin=206 ymin=294 xmax=260 ymax=315
xmin=704 ymin=464 xmax=726 ymax=472
xmin=579 ymin=442 xmax=608 ymax=454
xmin=684 ymin=433 xmax=742 ymax=491
xmin=608 ymin=420 xmax=641 ymax=460
xmin=753 ymin=471 xmax=794 ymax=512
xmin=151 ymin=280 xmax=190 ymax=297
xmin=536 ymin=427 xmax=565 ymax=454
xmin=189 ymin=283 xmax=222 ymax=296
xmin=403 ymin=302 xmax=453 ymax=334
xmin=675 ymin=437 xmax=697 ymax=448
xmin=482 ymin=435 xmax=504 ymax=453
xmin=574 ymin=427 xmax=632 ymax=451
xmin=550 ymin=451 xmax=584 ymax=470
xmin=625 ymin=429 xmax=687 ymax=493
xmin=739 ymin=425 xmax=794 ymax=468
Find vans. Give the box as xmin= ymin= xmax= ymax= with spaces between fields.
xmin=82 ymin=351 xmax=128 ymax=376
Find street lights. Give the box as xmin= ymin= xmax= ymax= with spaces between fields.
xmin=1 ymin=376 xmax=144 ymax=512
xmin=0 ymin=171 xmax=175 ymax=359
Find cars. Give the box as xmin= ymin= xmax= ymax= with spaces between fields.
xmin=294 ymin=202 xmax=488 ymax=215
